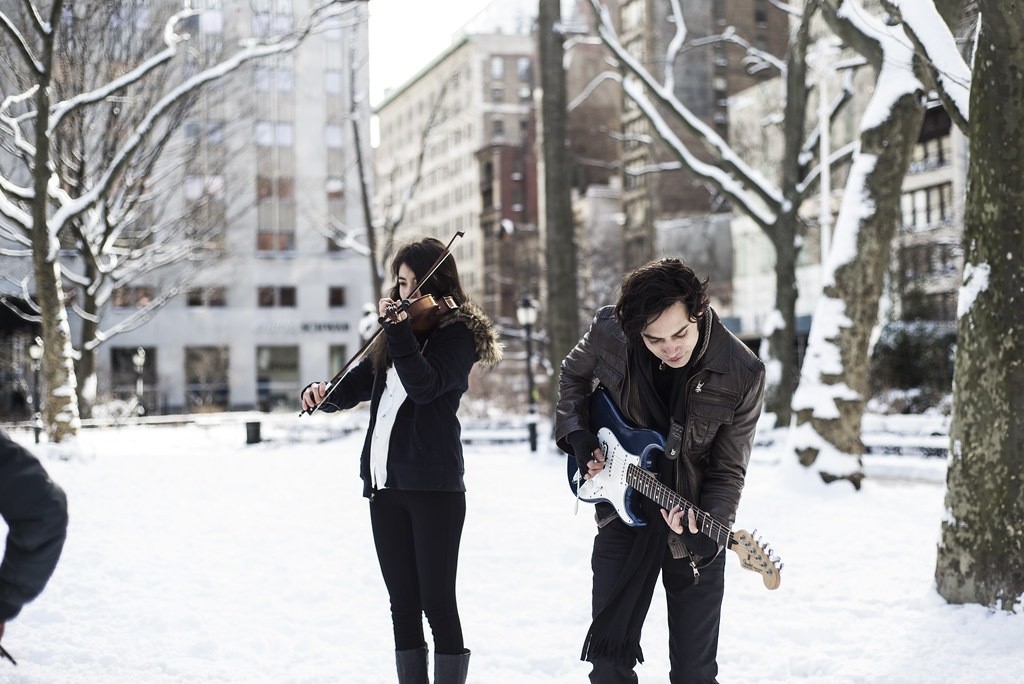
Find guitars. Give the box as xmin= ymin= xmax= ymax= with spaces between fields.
xmin=566 ymin=386 xmax=785 ymax=590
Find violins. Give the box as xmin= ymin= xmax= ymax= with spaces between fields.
xmin=378 ymin=293 xmax=459 ymax=332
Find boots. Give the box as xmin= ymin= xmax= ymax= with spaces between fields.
xmin=434 ymin=648 xmax=471 ymax=684
xmin=395 ymin=641 xmax=429 ymax=684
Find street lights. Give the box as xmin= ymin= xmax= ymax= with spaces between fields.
xmin=516 ymin=294 xmax=540 ymax=451
xmin=29 ymin=335 xmax=44 ymax=443
xmin=131 ymin=350 xmax=146 ymax=419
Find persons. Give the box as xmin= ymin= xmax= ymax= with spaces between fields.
xmin=554 ymin=257 xmax=766 ymax=684
xmin=0 ymin=430 xmax=68 ymax=666
xmin=300 ymin=237 xmax=504 ymax=684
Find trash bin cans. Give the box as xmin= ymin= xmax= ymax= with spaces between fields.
xmin=246 ymin=421 xmax=261 ymax=444
